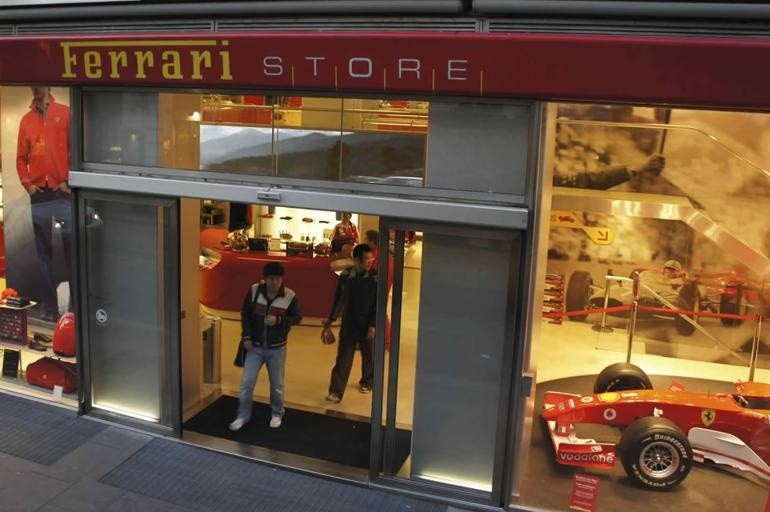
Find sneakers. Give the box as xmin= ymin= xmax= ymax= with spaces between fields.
xmin=325 ymin=393 xmax=341 ymax=403
xmin=269 ymin=415 xmax=283 ymax=429
xmin=360 ymin=386 xmax=369 ymax=393
xmin=41 ymin=310 xmax=58 ymax=323
xmin=29 ymin=340 xmax=47 ymax=351
xmin=34 ymin=334 xmax=52 ymax=342
xmin=228 ymin=416 xmax=245 ymax=432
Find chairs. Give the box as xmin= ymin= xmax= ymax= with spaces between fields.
xmin=248 ymin=238 xmax=313 ymax=257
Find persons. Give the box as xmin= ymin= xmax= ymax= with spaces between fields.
xmin=363 ymin=227 xmax=395 ymax=354
xmin=318 ymin=243 xmax=377 ymax=403
xmin=327 ymin=212 xmax=359 ymax=254
xmin=13 ymin=86 xmax=78 ymax=276
xmin=224 ymin=258 xmax=302 ymax=434
xmin=407 ymin=231 xmax=417 ymax=243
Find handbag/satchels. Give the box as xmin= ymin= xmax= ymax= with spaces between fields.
xmin=26 ymin=356 xmax=79 ymax=392
xmin=234 ymin=341 xmax=247 ymax=367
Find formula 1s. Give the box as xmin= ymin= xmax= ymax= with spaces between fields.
xmin=537 ymin=360 xmax=769 ymax=494
xmin=563 ymin=254 xmax=749 ymax=335
